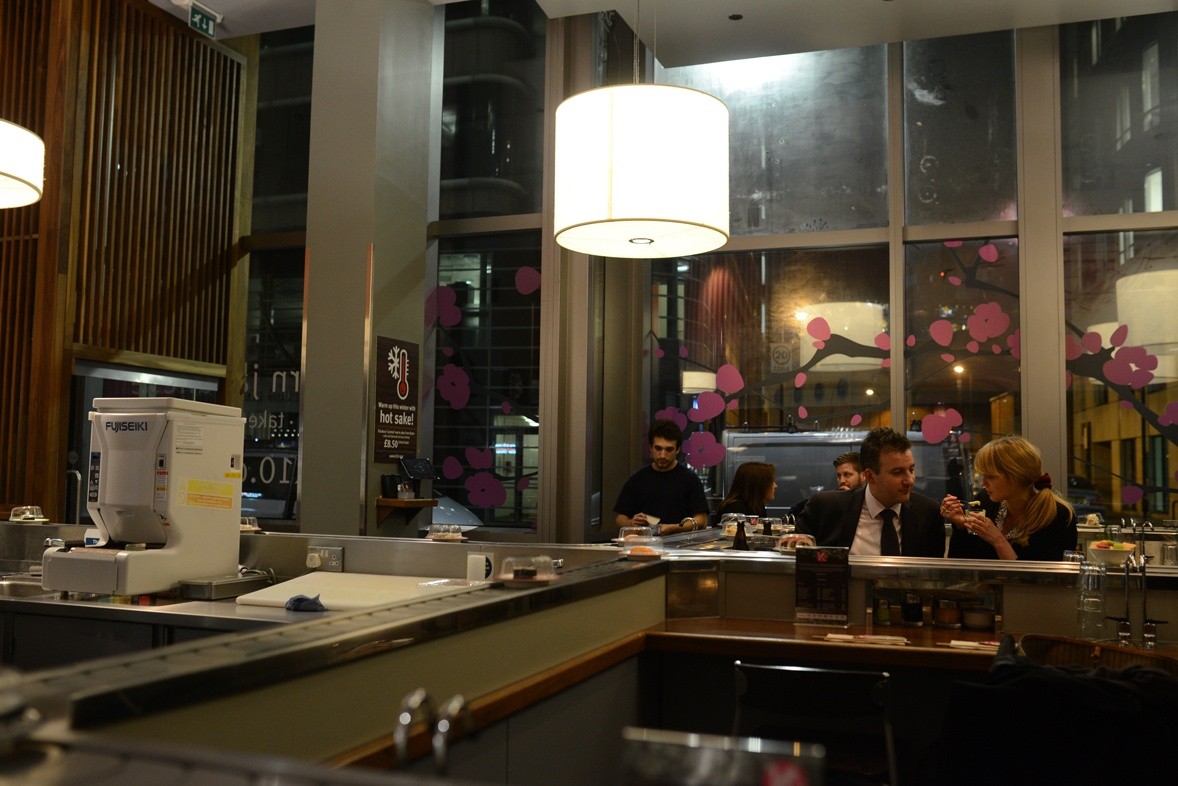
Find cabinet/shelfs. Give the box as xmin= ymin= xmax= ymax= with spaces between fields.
xmin=988 ymin=389 xmax=1021 ymax=441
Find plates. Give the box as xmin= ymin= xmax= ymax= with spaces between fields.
xmin=1075 ymin=524 xmax=1105 ymax=529
xmin=617 ymin=551 xmax=669 ymax=561
xmin=610 ymin=537 xmax=660 ymax=546
xmin=717 ymin=523 xmax=818 ymax=554
xmin=240 ymin=527 xmax=262 ymax=534
xmin=494 ymin=573 xmax=558 ymax=590
xmin=425 ymin=537 xmax=469 ymax=543
xmin=9 ymin=518 xmax=49 ymax=525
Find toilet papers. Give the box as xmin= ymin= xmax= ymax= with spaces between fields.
xmin=466 ymin=555 xmax=486 ymax=580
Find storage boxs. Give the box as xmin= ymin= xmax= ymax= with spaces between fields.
xmin=177 ymin=573 xmax=270 ymax=601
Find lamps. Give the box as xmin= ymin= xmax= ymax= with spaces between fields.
xmin=1085 ymin=321 xmax=1178 ymax=389
xmin=683 ymin=369 xmax=718 ymax=395
xmin=0 ymin=117 xmax=47 ymax=210
xmin=1116 ymin=268 xmax=1178 ymax=379
xmin=797 ymin=300 xmax=888 ymax=373
xmin=552 ymin=0 xmax=732 ymax=261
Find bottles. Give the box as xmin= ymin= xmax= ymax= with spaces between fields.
xmin=733 ymin=521 xmax=750 ymax=551
xmin=762 ymin=521 xmax=772 ymax=535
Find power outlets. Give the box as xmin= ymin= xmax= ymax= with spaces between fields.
xmin=307 ymin=546 xmax=343 ymax=572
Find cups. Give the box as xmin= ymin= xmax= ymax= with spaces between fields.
xmin=964 ymin=508 xmax=986 ymax=535
xmin=1075 ymin=561 xmax=1107 ymax=638
xmin=466 ymin=552 xmax=486 ymax=581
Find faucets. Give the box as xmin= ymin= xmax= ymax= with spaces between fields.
xmin=1131 ymin=522 xmax=1137 ymax=545
xmin=679 ymin=517 xmax=698 ymax=532
xmin=1103 ymin=555 xmax=1137 ymax=647
xmin=1140 ymin=521 xmax=1154 ymax=564
xmin=1120 ymin=518 xmax=1127 ymax=527
xmin=1129 ymin=518 xmax=1136 ymax=526
xmin=1138 ymin=555 xmax=1169 ymax=648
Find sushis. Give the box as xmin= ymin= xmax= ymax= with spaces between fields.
xmin=432 ymin=532 xmax=461 ymax=539
xmin=512 ymin=567 xmax=537 ymax=579
xmin=778 ymin=537 xmax=813 ymax=550
xmin=629 ymin=546 xmax=656 ymax=554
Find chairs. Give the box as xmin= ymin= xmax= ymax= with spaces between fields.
xmin=728 ymin=656 xmax=908 ymax=786
xmin=916 ymin=634 xmax=1178 ymax=786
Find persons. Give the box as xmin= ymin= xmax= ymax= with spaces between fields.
xmin=942 ymin=428 xmax=1085 ymax=559
xmin=712 ymin=461 xmax=779 ymax=538
xmin=612 ymin=416 xmax=710 ymax=532
xmin=787 ymin=426 xmax=946 ymax=559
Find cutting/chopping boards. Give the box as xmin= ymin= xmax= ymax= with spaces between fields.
xmin=236 ymin=572 xmax=471 ymax=609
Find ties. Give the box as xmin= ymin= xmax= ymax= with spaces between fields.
xmin=880 ymin=509 xmax=900 ymax=556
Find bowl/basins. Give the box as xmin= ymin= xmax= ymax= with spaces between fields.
xmin=1087 ymin=548 xmax=1134 ymax=567
xmin=962 ymin=610 xmax=994 ymax=631
